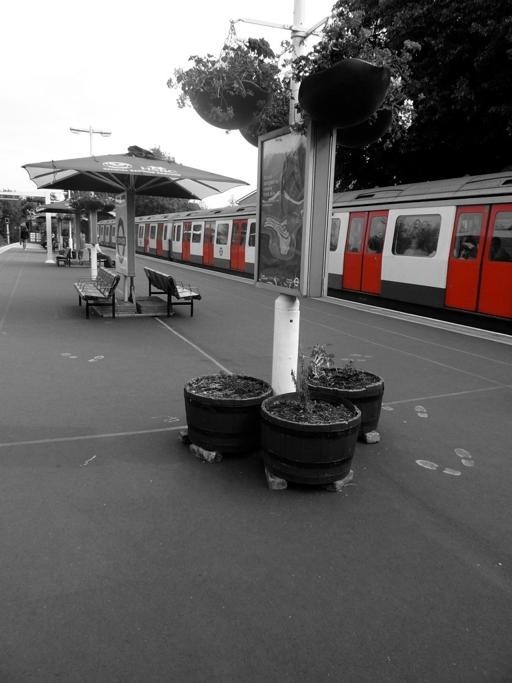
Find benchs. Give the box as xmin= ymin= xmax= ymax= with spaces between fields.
xmin=56 ymin=246 xmax=70 ymax=267
xmin=74 ymin=267 xmax=121 ymax=319
xmin=96 ymin=245 xmax=108 ymax=267
xmin=144 ymin=265 xmax=202 ymax=317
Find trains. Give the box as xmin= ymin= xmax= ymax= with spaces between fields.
xmin=97 ymin=172 xmax=512 ymax=324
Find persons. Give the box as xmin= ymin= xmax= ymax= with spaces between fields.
xmin=20 ymin=226 xmax=29 ymax=248
xmin=97 ymin=234 xmax=115 ymax=267
xmin=492 ymin=236 xmax=508 ymax=258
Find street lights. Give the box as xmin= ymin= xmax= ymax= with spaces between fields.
xmin=69 ymin=123 xmax=113 ymax=280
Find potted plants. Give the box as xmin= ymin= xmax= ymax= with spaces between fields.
xmin=182 ymin=343 xmax=386 ymax=493
